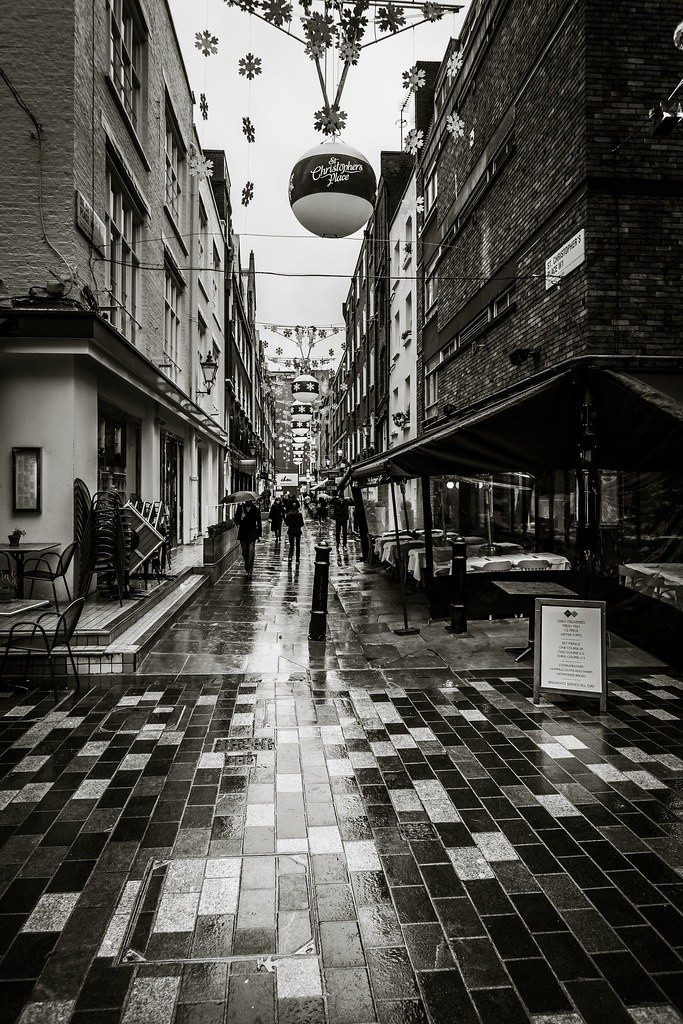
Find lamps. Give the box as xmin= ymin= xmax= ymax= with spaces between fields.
xmin=509 ymin=347 xmax=538 ymax=365
xmin=195 ymin=351 xmax=219 ymax=395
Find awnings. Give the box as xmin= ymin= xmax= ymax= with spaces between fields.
xmin=309 ymin=477 xmax=328 ymax=491
xmin=358 ymin=370 xmax=576 ymax=602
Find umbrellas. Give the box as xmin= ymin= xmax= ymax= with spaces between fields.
xmin=220 ymin=491 xmax=260 ymax=513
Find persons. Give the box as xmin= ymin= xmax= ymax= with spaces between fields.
xmin=306 ymin=493 xmax=354 ymax=551
xmin=234 ymin=501 xmax=262 ymax=576
xmin=268 ymin=494 xmax=304 ymax=564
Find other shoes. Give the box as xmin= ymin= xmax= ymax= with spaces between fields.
xmin=279 ymin=538 xmax=280 ymax=541
xmin=343 ymin=547 xmax=347 ymax=551
xmin=296 ymin=559 xmax=299 ymax=562
xmin=275 ymin=539 xmax=277 ymax=542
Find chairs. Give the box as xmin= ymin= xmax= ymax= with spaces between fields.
xmin=0 ymin=597 xmax=86 ymax=702
xmin=23 ymin=541 xmax=78 ymax=618
xmin=74 ymin=478 xmax=132 ymax=607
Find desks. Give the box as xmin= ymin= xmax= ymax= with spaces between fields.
xmin=0 ymin=543 xmax=61 ymax=601
xmin=119 ymin=500 xmax=166 ymax=576
xmin=374 ymin=529 xmax=572 ymax=619
xmin=492 ymin=580 xmax=580 ymax=663
